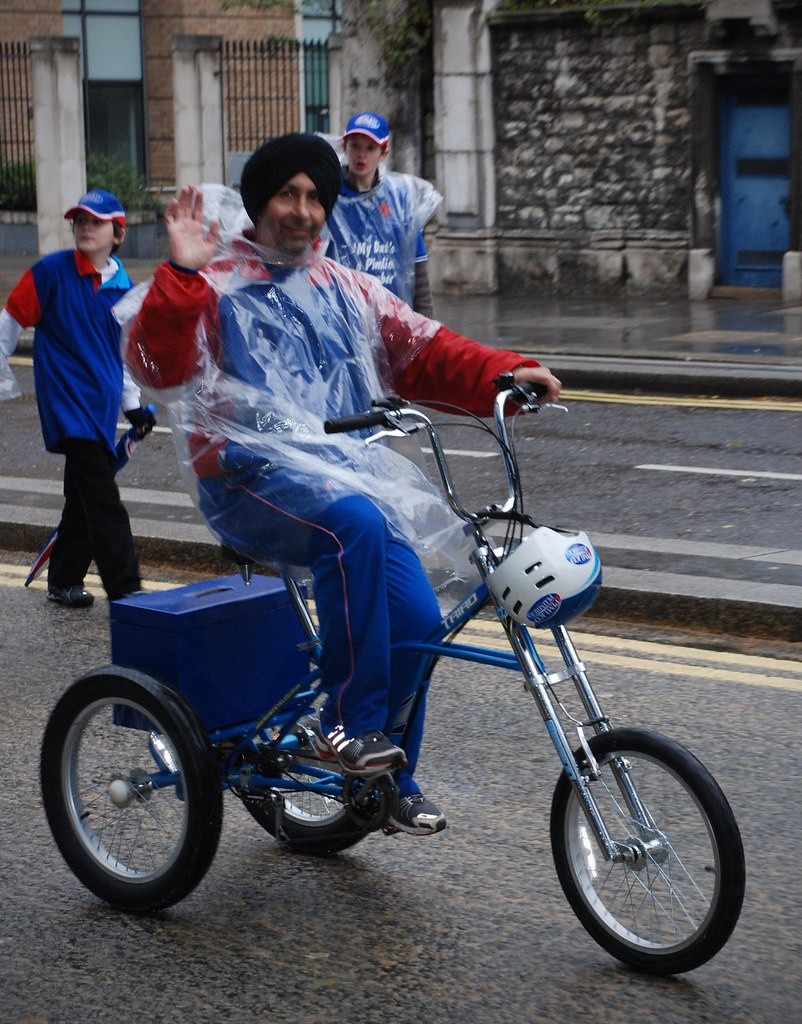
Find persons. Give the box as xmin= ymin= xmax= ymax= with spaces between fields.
xmin=323 ymin=112 xmax=445 ymax=320
xmin=1 ymin=191 xmax=156 ymax=607
xmin=127 ymin=132 xmax=563 ymax=835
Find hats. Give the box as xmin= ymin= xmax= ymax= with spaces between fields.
xmin=65 ymin=191 xmax=128 ymax=228
xmin=340 ymin=113 xmax=391 ymax=144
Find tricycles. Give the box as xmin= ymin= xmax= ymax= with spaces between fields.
xmin=40 ymin=371 xmax=746 ymax=976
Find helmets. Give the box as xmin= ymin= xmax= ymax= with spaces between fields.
xmin=483 ymin=526 xmax=604 ymax=630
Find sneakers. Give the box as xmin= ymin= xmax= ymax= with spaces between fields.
xmin=387 ymin=793 xmax=446 ymax=836
xmin=317 ymin=721 xmax=408 ymax=775
xmin=46 ymin=584 xmax=94 ymax=606
